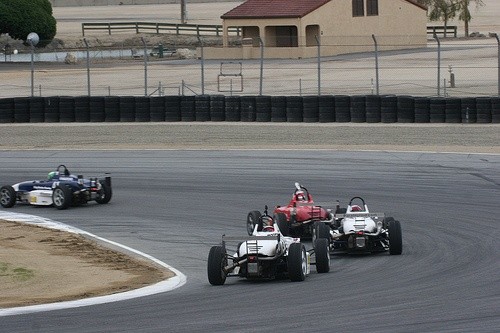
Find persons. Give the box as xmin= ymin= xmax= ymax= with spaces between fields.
xmin=47 ymin=171 xmax=59 ymax=182
xmin=351 ymin=205 xmax=363 ymax=212
xmin=261 ymin=226 xmax=273 ymax=233
xmin=296 ymin=191 xmax=305 ymax=201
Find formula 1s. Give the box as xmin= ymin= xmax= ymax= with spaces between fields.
xmin=1 ymin=164 xmax=113 ymax=209
xmin=206 ymin=215 xmax=331 ymax=286
xmin=245 ymin=186 xmax=333 ymax=237
xmin=313 ymin=197 xmax=403 ymax=256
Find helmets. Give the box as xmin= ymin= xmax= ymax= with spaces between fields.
xmin=48 ymin=171 xmax=59 ymax=180
xmin=293 ymin=190 xmax=305 ymax=201
xmin=262 ymin=226 xmax=274 ymax=233
xmin=350 ymin=205 xmax=362 ymax=212
